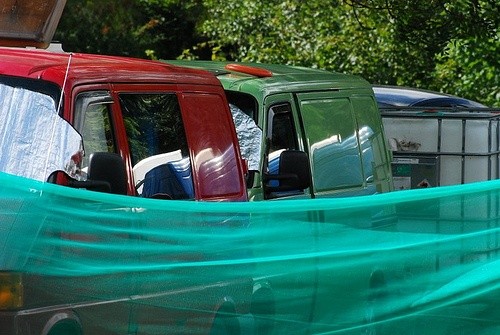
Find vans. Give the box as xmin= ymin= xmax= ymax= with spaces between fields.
xmin=0 ymin=46 xmax=255 ymax=335
xmin=156 ymin=57 xmax=395 ymax=335
